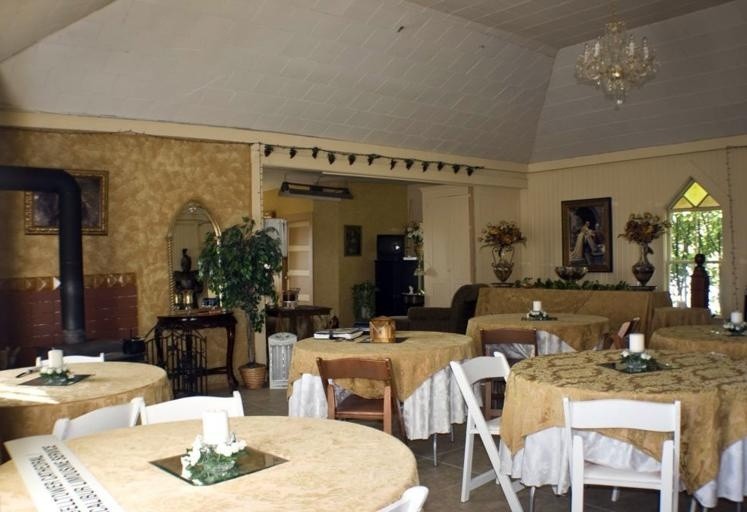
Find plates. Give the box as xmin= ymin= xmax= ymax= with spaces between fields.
xmin=629 ymin=285 xmax=656 ymax=291
xmin=490 ymin=282 xmax=515 ymax=288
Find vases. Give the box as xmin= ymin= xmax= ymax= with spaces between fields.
xmin=412 ymin=244 xmax=424 ymax=294
xmin=491 ymin=245 xmax=515 ymax=284
xmin=632 ymin=242 xmax=656 ymax=287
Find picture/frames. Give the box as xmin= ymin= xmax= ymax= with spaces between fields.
xmin=24 ymin=169 xmax=109 ymax=237
xmin=560 ymin=197 xmax=614 ymax=274
xmin=343 ymin=225 xmax=363 ymax=258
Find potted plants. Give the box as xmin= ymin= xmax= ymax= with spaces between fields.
xmin=199 ymin=216 xmax=285 ymax=390
xmin=351 ymin=280 xmax=379 ymax=322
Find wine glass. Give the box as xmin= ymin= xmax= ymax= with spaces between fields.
xmin=174 ymin=294 xmax=182 ymax=311
xmin=183 ymin=289 xmax=194 ymax=311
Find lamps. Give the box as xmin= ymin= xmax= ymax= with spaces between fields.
xmin=573 ymin=4 xmax=660 ymax=111
xmin=278 ymin=181 xmax=354 ymax=202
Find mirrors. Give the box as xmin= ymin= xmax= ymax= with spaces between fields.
xmin=165 ymin=198 xmax=227 ymax=314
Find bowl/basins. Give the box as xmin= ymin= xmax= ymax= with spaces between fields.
xmin=554 ymin=265 xmax=588 ymax=280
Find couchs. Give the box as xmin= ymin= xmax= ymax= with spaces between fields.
xmin=389 ymin=283 xmax=489 ymax=336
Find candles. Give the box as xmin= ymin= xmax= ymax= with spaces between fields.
xmin=628 ymin=332 xmax=645 ymax=354
xmin=202 ymin=408 xmax=229 ymax=446
xmin=532 ymin=301 xmax=542 ymax=313
xmin=47 ymin=347 xmax=64 ymax=370
xmin=729 ymin=311 xmax=744 ymax=325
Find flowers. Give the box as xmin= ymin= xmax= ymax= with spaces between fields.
xmin=615 ymin=212 xmax=671 ymax=246
xmin=402 ymin=220 xmax=426 ymax=244
xmin=477 ymin=220 xmax=528 ymax=259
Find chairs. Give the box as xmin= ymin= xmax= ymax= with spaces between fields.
xmin=611 ymin=317 xmax=641 ymax=351
xmin=449 ymin=351 xmax=525 ymax=512
xmin=35 ymin=353 xmax=105 ymax=366
xmin=316 ymin=357 xmax=407 ymax=443
xmin=562 ymin=398 xmax=683 ymax=512
xmin=2 ymin=397 xmax=141 ymax=460
xmin=370 ymin=485 xmax=429 ymax=512
xmin=139 ymin=390 xmax=245 ymax=426
xmin=479 ymin=327 xmax=539 ymax=421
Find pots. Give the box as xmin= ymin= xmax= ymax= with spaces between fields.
xmin=122 ymin=335 xmax=146 ymax=356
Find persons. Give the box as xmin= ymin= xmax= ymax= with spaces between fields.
xmin=173 ymin=248 xmax=203 ymax=310
xmin=570 ymin=221 xmax=590 ymax=256
